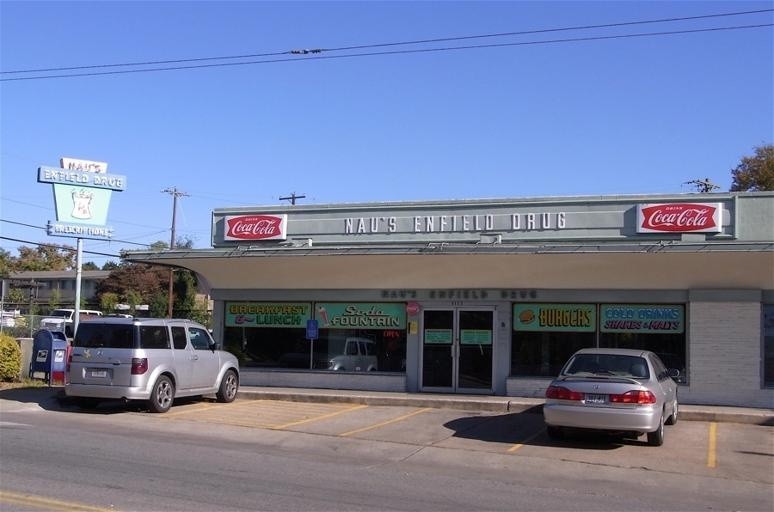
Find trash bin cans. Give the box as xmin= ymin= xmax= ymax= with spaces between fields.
xmin=29 ymin=329 xmax=68 ymax=387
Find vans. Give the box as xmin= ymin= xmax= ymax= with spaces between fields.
xmin=40 ymin=308 xmax=104 ymax=329
xmin=62 ymin=316 xmax=241 ymax=413
xmin=326 ymin=333 xmax=379 ymax=373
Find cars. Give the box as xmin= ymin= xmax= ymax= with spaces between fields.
xmin=542 ymin=347 xmax=680 ymax=447
